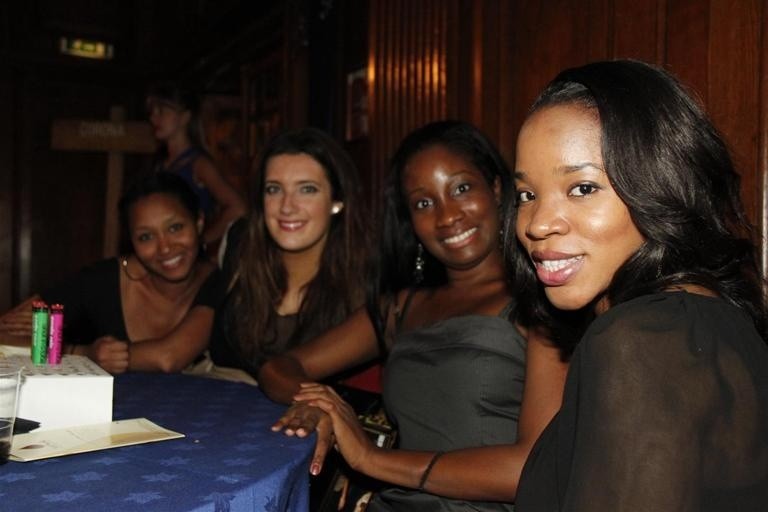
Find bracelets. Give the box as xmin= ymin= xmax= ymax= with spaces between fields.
xmin=69 ymin=343 xmax=79 ymax=356
xmin=197 ymin=237 xmax=210 ymax=260
xmin=417 ymin=450 xmax=445 ymax=493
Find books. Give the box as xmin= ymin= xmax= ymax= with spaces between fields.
xmin=0 ymin=416 xmax=187 ymax=467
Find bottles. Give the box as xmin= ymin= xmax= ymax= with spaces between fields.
xmin=31 ymin=299 xmax=65 ymax=367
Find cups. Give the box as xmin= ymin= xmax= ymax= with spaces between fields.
xmin=0 ymin=360 xmax=26 ymax=466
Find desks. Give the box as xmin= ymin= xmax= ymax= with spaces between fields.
xmin=1 ymin=369 xmax=316 ymax=511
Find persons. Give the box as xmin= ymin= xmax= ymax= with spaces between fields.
xmin=0 ymin=172 xmax=227 ymax=374
xmin=269 ymin=116 xmax=577 ymax=512
xmin=505 ymin=54 xmax=766 ymax=512
xmin=183 ymin=120 xmax=392 ymax=388
xmin=135 ymin=71 xmax=253 ymax=252
